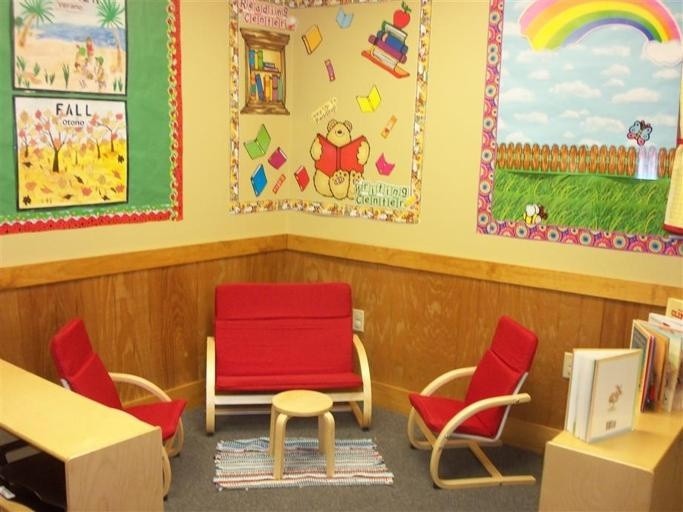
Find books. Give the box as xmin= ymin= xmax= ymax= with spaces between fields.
xmin=561 ymin=295 xmax=683 ymax=446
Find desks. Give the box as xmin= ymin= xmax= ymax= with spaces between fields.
xmin=0 ymin=356 xmax=163 ymax=511
xmin=537 ymin=392 xmax=683 ymax=512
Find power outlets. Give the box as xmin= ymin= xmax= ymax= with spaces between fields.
xmin=561 ymin=352 xmax=574 ymax=378
